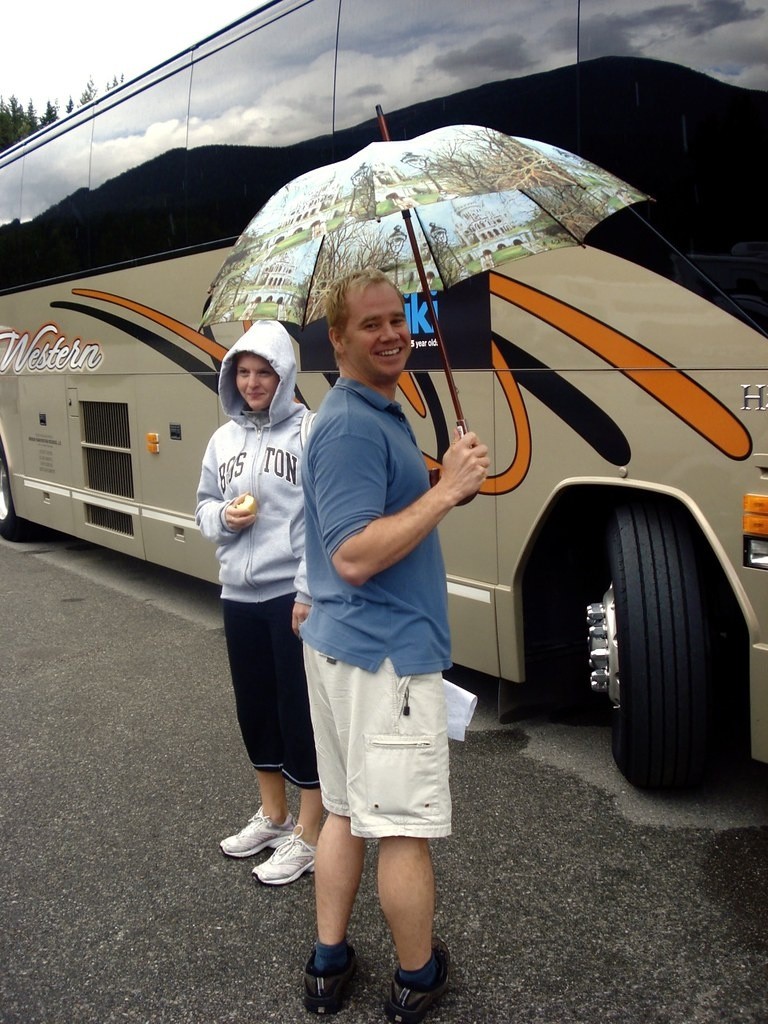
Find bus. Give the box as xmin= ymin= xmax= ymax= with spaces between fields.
xmin=1 ymin=0 xmax=767 ymax=804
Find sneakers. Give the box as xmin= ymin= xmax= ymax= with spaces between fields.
xmin=385 ymin=938 xmax=450 ymax=1024
xmin=220 ymin=805 xmax=296 ymax=858
xmin=251 ymin=825 xmax=316 ymax=886
xmin=303 ymin=943 xmax=357 ymax=1014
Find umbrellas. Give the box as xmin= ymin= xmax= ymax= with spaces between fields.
xmin=194 ymin=100 xmax=653 ymax=508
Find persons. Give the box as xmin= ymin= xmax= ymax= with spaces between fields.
xmin=298 ymin=264 xmax=492 ymax=1019
xmin=192 ymin=317 xmax=326 ymax=887
